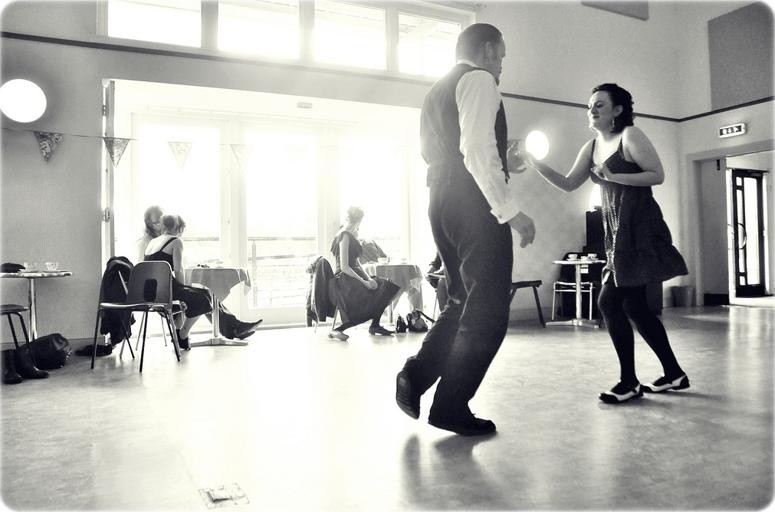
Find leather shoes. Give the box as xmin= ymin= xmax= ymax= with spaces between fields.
xmin=598 ymin=381 xmax=644 ymax=405
xmin=427 ymin=397 xmax=497 ymax=437
xmin=394 ymin=360 xmax=422 ymax=420
xmin=231 ymin=318 xmax=264 ymax=341
xmin=640 ymin=372 xmax=691 ymax=394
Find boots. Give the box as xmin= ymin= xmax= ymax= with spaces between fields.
xmin=0 ymin=348 xmax=23 ymax=385
xmin=13 ymin=347 xmax=51 ymax=380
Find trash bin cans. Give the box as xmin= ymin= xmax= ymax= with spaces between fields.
xmin=672 ymin=285 xmax=692 ymax=307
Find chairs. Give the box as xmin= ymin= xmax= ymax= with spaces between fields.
xmin=306 ymin=256 xmax=360 ymax=334
xmin=108 ymin=256 xmax=183 ymax=359
xmin=93 ymin=261 xmax=183 ymax=374
xmin=549 ymin=251 xmax=597 ymax=319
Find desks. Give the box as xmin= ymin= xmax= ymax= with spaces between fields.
xmin=176 ymin=265 xmax=252 ymax=347
xmin=0 ymin=269 xmax=73 ymax=343
xmin=550 ymin=258 xmax=607 ymax=319
xmin=352 ymin=263 xmax=426 ymax=323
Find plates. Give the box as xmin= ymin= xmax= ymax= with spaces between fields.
xmin=567 ymin=259 xmax=581 ymax=262
xmin=44 ymin=270 xmax=60 ymax=273
xmin=21 ymin=269 xmax=38 ymax=272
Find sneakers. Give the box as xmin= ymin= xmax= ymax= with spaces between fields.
xmin=171 ymin=328 xmax=191 ymax=351
xmin=74 ymin=343 xmax=114 ymax=357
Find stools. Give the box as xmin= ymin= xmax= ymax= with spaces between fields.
xmin=430 ymin=288 xmax=445 ymax=322
xmin=507 ymin=280 xmax=547 ymax=328
xmin=0 ymin=303 xmax=39 ymax=367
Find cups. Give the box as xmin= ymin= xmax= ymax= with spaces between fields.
xmin=46 ymin=263 xmax=58 ymax=270
xmin=23 ymin=260 xmax=37 ymax=268
xmin=378 ymin=257 xmax=389 ymax=264
xmin=569 ymin=254 xmax=582 ymax=260
xmin=582 ymin=252 xmax=598 ymax=261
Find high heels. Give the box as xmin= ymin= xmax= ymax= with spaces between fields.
xmin=368 ymin=325 xmax=395 ymax=336
xmin=327 ymin=329 xmax=350 ymax=341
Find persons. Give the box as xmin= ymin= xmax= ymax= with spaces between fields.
xmin=426 ymin=251 xmax=449 ymax=314
xmin=141 ymin=206 xmax=264 ymax=340
xmin=328 ymin=208 xmax=402 ymax=340
xmin=339 ymin=223 xmax=424 ymax=325
xmin=389 ymin=21 xmax=538 ymax=435
xmin=145 ymin=213 xmax=215 ymax=352
xmin=515 ymin=80 xmax=691 ymax=405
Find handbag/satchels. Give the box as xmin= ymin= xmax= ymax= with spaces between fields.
xmin=406 ymin=310 xmax=429 ymax=333
xmin=358 ymin=238 xmax=388 ymax=266
xmin=395 ymin=315 xmax=407 ymax=334
xmin=18 ymin=332 xmax=73 ymax=371
